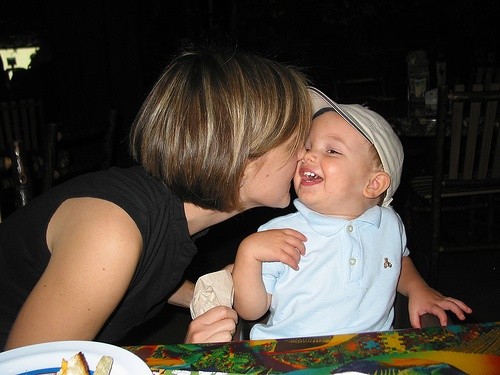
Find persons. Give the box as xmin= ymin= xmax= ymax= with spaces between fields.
xmin=0 ymin=52 xmax=314 ymax=352
xmin=232 ymin=86 xmax=472 ymax=341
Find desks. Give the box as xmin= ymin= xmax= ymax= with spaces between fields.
xmin=352 ymin=94 xmax=500 ymax=261
xmin=120 ymin=322 xmax=500 ymax=375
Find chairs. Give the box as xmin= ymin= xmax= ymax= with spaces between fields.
xmin=0 ymin=99 xmax=57 ymax=189
xmin=332 ymin=65 xmax=500 ymax=287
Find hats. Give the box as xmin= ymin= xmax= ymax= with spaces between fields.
xmin=306 ymin=84 xmax=404 ymax=208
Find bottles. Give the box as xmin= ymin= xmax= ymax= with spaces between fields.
xmin=8 ymin=141 xmax=31 ymax=210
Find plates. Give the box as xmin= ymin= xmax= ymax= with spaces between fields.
xmin=0 ymin=340 xmax=154 ymax=375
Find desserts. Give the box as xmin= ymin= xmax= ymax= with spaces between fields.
xmin=56 ymin=351 xmax=91 ymax=375
